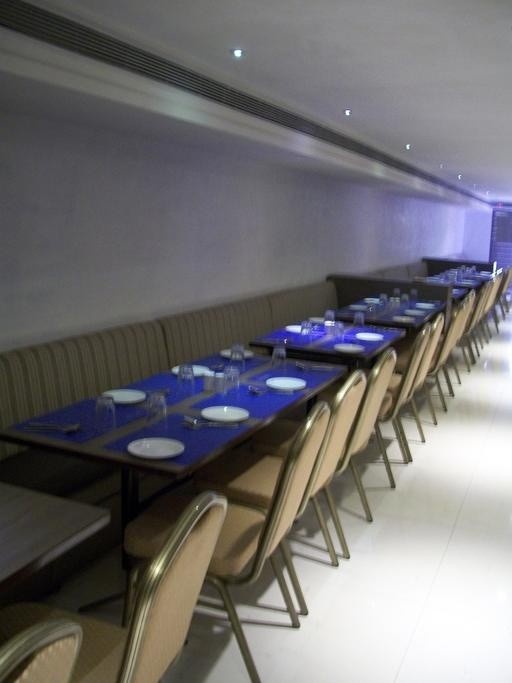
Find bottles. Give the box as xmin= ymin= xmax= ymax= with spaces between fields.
xmin=214 ymin=373 xmax=225 ymax=393
xmin=203 ymin=370 xmax=215 ymax=391
xmin=395 ymin=297 xmax=400 ymax=308
xmin=389 ymin=296 xmax=395 ymax=307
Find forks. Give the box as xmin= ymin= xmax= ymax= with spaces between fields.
xmin=183 ymin=413 xmax=202 ymax=425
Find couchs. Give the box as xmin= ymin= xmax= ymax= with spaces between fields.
xmin=0 ymin=316 xmax=171 ymax=465
xmin=156 ymin=294 xmax=273 ymax=374
xmin=270 ymin=277 xmax=339 ymax=331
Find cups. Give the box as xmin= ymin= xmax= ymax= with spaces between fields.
xmin=177 ymin=364 xmax=196 ymax=390
xmin=333 ymin=321 xmax=346 ymax=343
xmin=270 ymin=345 xmax=288 ymax=371
xmin=366 ymin=302 xmax=376 ymax=321
xmin=300 ymin=320 xmax=314 ymax=336
xmin=353 ymin=311 xmax=365 ymax=329
xmin=95 ymin=395 xmax=116 ymax=431
xmin=409 ymin=287 xmax=419 ymax=301
xmin=439 ymin=264 xmax=477 ymax=284
xmin=231 ymin=342 xmax=246 ymax=372
xmin=392 ymin=287 xmax=401 ymax=297
xmin=145 ymin=392 xmax=168 ymax=424
xmin=323 ymin=309 xmax=336 ymax=327
xmin=224 ymin=365 xmax=240 ymax=395
xmin=400 ymin=293 xmax=410 ymax=307
xmin=379 ymin=292 xmax=387 ymax=310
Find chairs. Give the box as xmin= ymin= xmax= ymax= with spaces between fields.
xmin=0 ymin=617 xmax=84 ymax=683
xmin=226 ymin=368 xmax=371 ymax=569
xmin=0 ymin=488 xmax=230 ymax=683
xmin=118 ymin=397 xmax=333 ymax=683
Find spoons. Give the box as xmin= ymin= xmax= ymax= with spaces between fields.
xmin=183 ymin=422 xmax=242 ymax=432
xmin=23 ymin=422 xmax=80 ymax=434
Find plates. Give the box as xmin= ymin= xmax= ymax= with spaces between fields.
xmin=285 ymin=324 xmax=309 ymax=334
xmin=126 ymin=436 xmax=186 ymax=460
xmin=363 ymin=297 xmax=380 ymax=304
xmin=348 ymin=304 xmax=367 ymax=311
xmin=404 ymin=310 xmax=426 ymax=316
xmin=452 ymin=270 xmax=493 ymax=295
xmin=355 ymin=332 xmax=384 ymax=342
xmin=333 ymin=343 xmax=366 ymax=354
xmin=102 ymin=388 xmax=147 ymax=404
xmin=415 ymin=302 xmax=436 ymax=309
xmin=171 ymin=363 xmax=210 ymax=378
xmin=219 ymin=348 xmax=254 ymax=360
xmin=309 ymin=316 xmax=326 ymax=323
xmin=199 ymin=405 xmax=251 ymax=424
xmin=265 ymin=375 xmax=307 ymax=390
xmin=392 ymin=315 xmax=416 ymax=324
